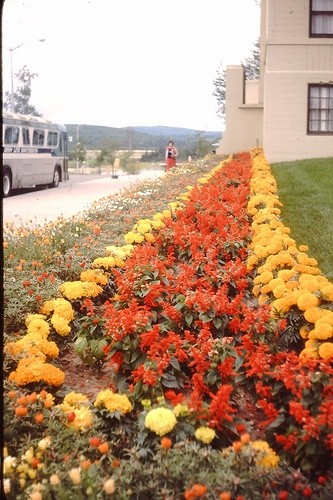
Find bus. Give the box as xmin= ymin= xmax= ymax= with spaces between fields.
xmin=2 ymin=112 xmax=69 ymax=197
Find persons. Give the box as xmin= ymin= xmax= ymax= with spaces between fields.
xmin=165 ymin=141 xmax=180 ymax=170
xmin=36 ymin=133 xmax=44 ymax=145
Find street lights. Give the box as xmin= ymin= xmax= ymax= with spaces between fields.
xmin=1 ymin=38 xmax=47 ymax=113
